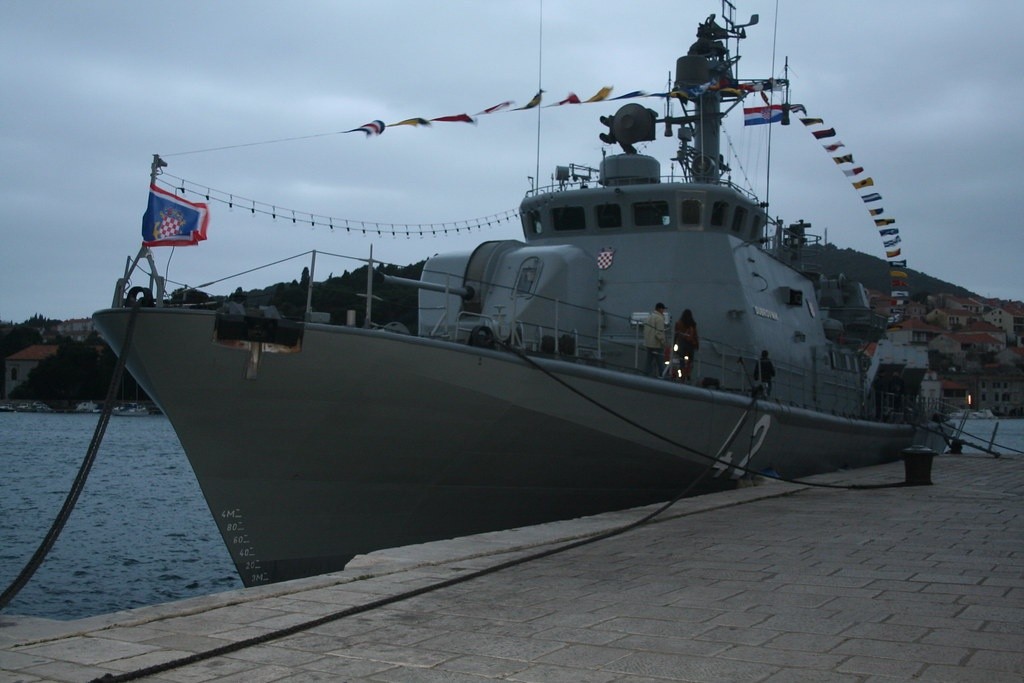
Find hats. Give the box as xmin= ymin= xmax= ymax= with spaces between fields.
xmin=656 ymin=302 xmax=668 ymax=309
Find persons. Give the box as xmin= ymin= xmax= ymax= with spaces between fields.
xmin=753 ymin=349 xmax=776 ymax=395
xmin=644 ymin=303 xmax=669 ymax=376
xmin=672 ymin=309 xmax=700 ymax=381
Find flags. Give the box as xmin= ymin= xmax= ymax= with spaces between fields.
xmin=823 ymin=141 xmax=845 ymax=152
xmin=880 ymin=228 xmax=909 ymax=313
xmin=843 ymin=167 xmax=864 ymax=177
xmin=655 ymin=77 xmax=784 ymax=98
xmin=611 ymin=89 xmax=647 ymax=101
xmin=812 ymin=127 xmax=836 ymax=140
xmin=869 ymin=208 xmax=884 ymax=216
xmin=833 ymin=153 xmax=855 ymax=164
xmin=587 ymin=86 xmax=611 ymax=104
xmin=874 ymin=219 xmax=896 ymax=226
xmin=799 ymin=117 xmax=825 ymax=126
xmin=479 ymin=99 xmax=512 ymax=117
xmin=790 ymin=104 xmax=806 ymax=115
xmin=743 ymin=104 xmax=784 ymax=126
xmin=852 ymin=177 xmax=874 ymax=190
xmin=389 ymin=118 xmax=430 ymax=129
xmin=861 ymin=193 xmax=882 ymax=203
xmin=520 ymin=90 xmax=543 ymax=112
xmin=552 ymin=93 xmax=582 ymax=105
xmin=433 ymin=113 xmax=474 ymax=124
xmin=142 ymin=187 xmax=210 ymax=247
xmin=355 ymin=121 xmax=385 ymax=137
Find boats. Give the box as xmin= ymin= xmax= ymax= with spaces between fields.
xmin=92 ymin=0 xmax=968 ymax=588
